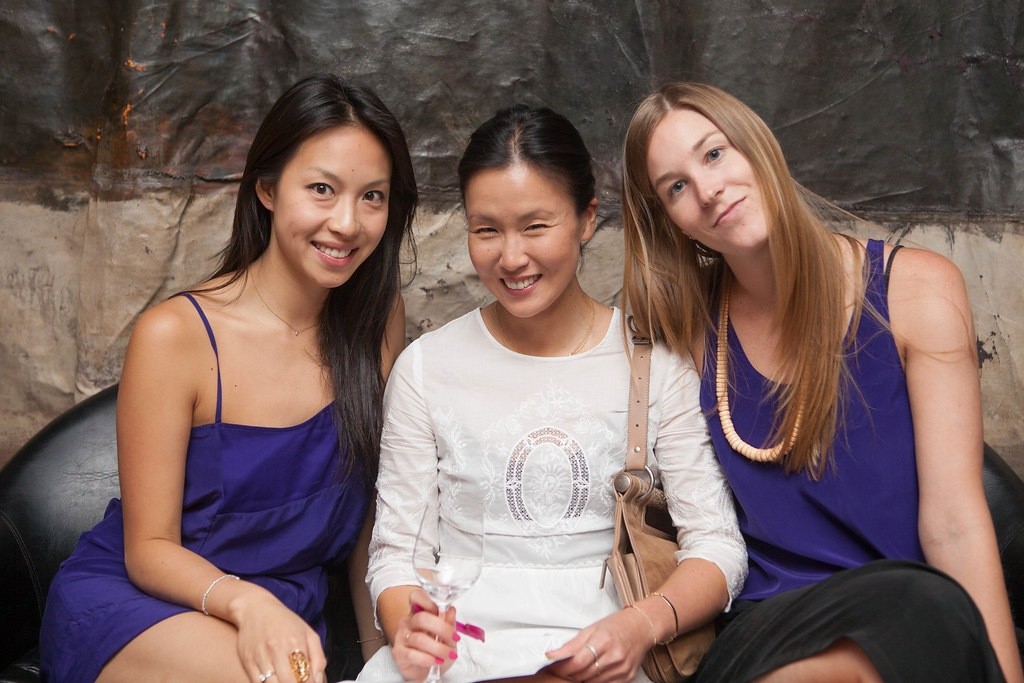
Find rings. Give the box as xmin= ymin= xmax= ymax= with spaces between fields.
xmin=258 ymin=670 xmax=276 ymax=683
xmin=594 ymin=661 xmax=601 ymax=675
xmin=289 ymin=651 xmax=311 ymax=683
xmin=586 ymin=644 xmax=599 ymax=660
xmin=405 ymin=630 xmax=412 ymax=648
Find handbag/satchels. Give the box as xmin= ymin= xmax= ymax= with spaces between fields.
xmin=601 ymin=336 xmax=718 ymax=683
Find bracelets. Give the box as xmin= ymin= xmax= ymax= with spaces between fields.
xmin=623 ymin=604 xmax=657 ymax=648
xmin=202 ymin=574 xmax=241 ymax=617
xmin=357 ymin=635 xmax=386 ymax=643
xmin=646 ymin=592 xmax=679 ymax=645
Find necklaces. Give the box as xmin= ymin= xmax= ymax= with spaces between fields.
xmin=716 ymin=254 xmax=818 ymax=462
xmin=494 ymin=293 xmax=596 ymax=357
xmin=250 ymin=270 xmax=321 ymax=337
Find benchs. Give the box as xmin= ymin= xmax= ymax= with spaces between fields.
xmin=0 ymin=383 xmax=1024 ymax=683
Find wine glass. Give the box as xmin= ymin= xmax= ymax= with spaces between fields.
xmin=412 ymin=505 xmax=483 ymax=683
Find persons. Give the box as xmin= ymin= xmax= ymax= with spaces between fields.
xmin=41 ymin=73 xmax=420 ymax=683
xmin=620 ymin=82 xmax=1024 ymax=683
xmin=355 ymin=103 xmax=751 ymax=683
